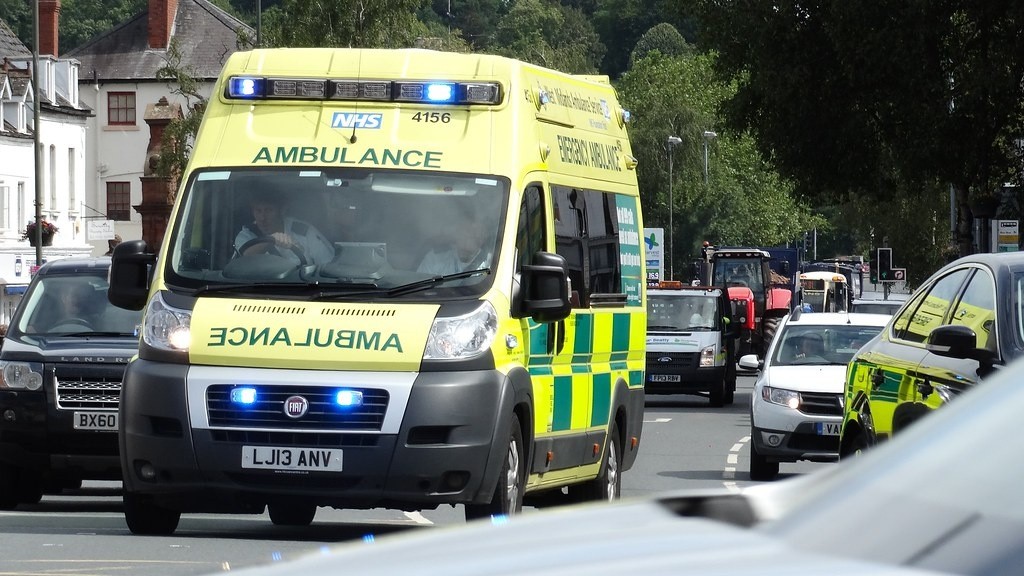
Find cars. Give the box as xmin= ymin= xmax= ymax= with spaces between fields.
xmin=851 ymin=296 xmax=906 ymax=320
xmin=839 ymin=252 xmax=1023 ymax=465
xmin=800 ymin=252 xmax=869 ymax=273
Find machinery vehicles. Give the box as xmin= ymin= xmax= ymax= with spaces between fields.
xmin=708 ymin=247 xmax=792 ymax=358
xmin=800 ymin=271 xmax=850 ymax=312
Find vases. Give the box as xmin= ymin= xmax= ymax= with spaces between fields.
xmin=28 ymin=232 xmax=53 ymax=246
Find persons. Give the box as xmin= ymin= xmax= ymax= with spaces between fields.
xmin=794 ymin=337 xmax=819 ymax=361
xmin=415 ymin=205 xmax=492 ymax=277
xmin=231 ymin=176 xmax=335 ymax=267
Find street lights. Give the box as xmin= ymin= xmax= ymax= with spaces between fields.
xmin=666 ymin=134 xmax=683 ymax=280
xmin=702 ymin=129 xmax=716 ymax=184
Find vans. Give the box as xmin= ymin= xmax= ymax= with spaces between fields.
xmin=110 ymin=43 xmax=650 ymax=532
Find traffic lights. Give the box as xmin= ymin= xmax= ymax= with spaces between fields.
xmin=867 ymin=248 xmax=893 ymax=283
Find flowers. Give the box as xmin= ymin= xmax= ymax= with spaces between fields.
xmin=26 ymin=220 xmax=57 ymax=234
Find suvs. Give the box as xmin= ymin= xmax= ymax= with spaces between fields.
xmin=645 ymin=277 xmax=736 ymax=407
xmin=0 ymin=255 xmax=156 ymax=502
xmin=739 ymin=301 xmax=905 ymax=479
